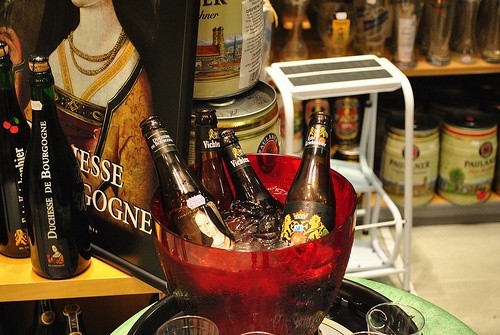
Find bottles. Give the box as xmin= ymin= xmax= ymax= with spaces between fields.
xmin=139 ymin=115 xmax=234 ymax=251
xmin=0 ymin=41 xmax=31 ymax=258
xmin=277 ymin=112 xmax=335 ymax=248
xmin=22 ymin=55 xmax=90 ymax=280
xmin=61 ymin=303 xmax=86 ymax=335
xmin=194 ymin=109 xmax=233 ymax=207
xmin=219 ymin=129 xmax=282 ymax=234
xmin=29 ymin=300 xmax=62 ymax=335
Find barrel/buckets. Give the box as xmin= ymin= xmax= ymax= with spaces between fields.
xmin=150 ymin=154 xmax=356 ymax=335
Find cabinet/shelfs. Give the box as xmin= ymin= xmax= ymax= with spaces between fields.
xmin=0 ymin=0 xmax=500 ymax=335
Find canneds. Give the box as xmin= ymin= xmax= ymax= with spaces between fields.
xmin=378 ymin=107 xmax=498 ymax=209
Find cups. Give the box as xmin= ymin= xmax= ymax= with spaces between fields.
xmin=154 ymin=315 xmax=219 ymax=335
xmin=273 ymin=0 xmax=500 ymax=71
xmin=366 ymin=301 xmax=425 ymax=335
